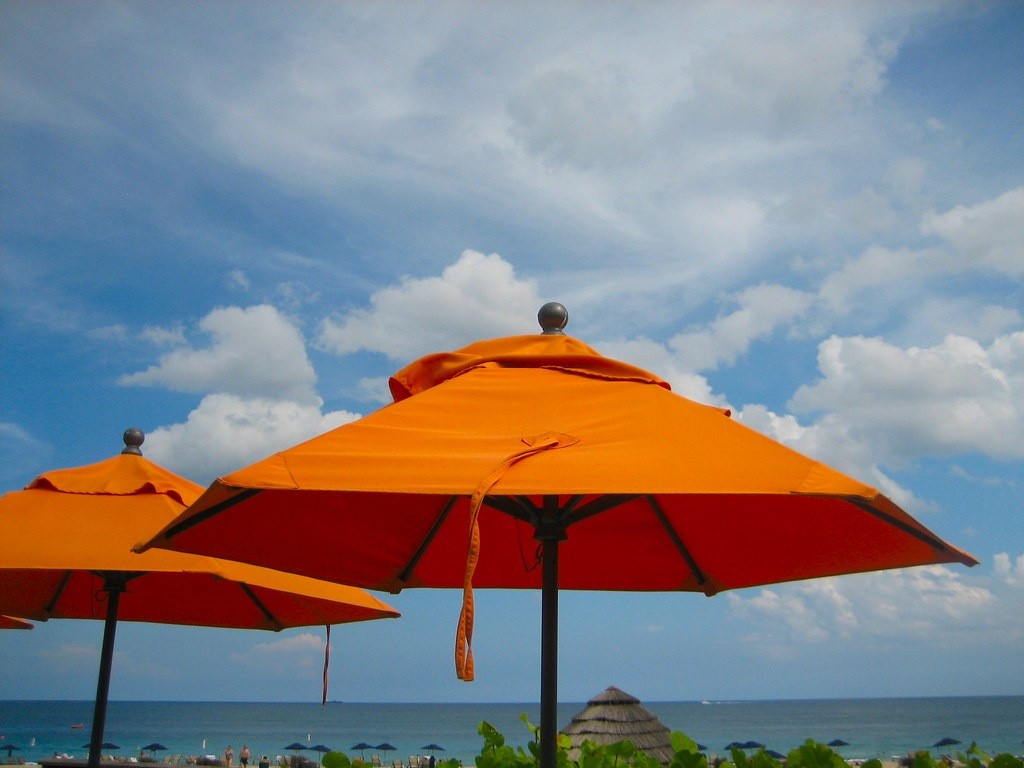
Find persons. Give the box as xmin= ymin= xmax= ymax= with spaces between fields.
xmin=239 ymin=744 xmax=250 ymax=768
xmin=225 ymin=745 xmax=233 ymax=768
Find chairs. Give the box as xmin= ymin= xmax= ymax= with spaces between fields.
xmin=372 ymin=754 xmax=383 ymax=768
xmin=393 ymin=755 xmax=434 ymax=768
xmin=276 ymin=755 xmax=317 ymax=768
xmin=163 ymin=754 xmax=195 ymax=765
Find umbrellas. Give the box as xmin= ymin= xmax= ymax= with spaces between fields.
xmin=827 ymin=739 xmax=849 ymax=754
xmin=375 ymin=743 xmax=397 ymax=761
xmin=421 ymin=744 xmax=446 ymax=753
xmin=933 ymin=737 xmax=963 ymax=756
xmin=81 ymin=742 xmax=120 ymax=754
xmin=308 ymin=745 xmax=333 ymax=761
xmin=283 ymin=742 xmax=308 ymax=756
xmin=351 ymin=743 xmax=374 ymax=760
xmin=0 ymin=426 xmax=401 ymax=768
xmin=131 ymin=299 xmax=980 ymax=768
xmin=142 ymin=743 xmax=168 ymax=759
xmin=697 ymin=740 xmax=786 ymax=759
xmin=0 ymin=744 xmax=21 ymax=756
